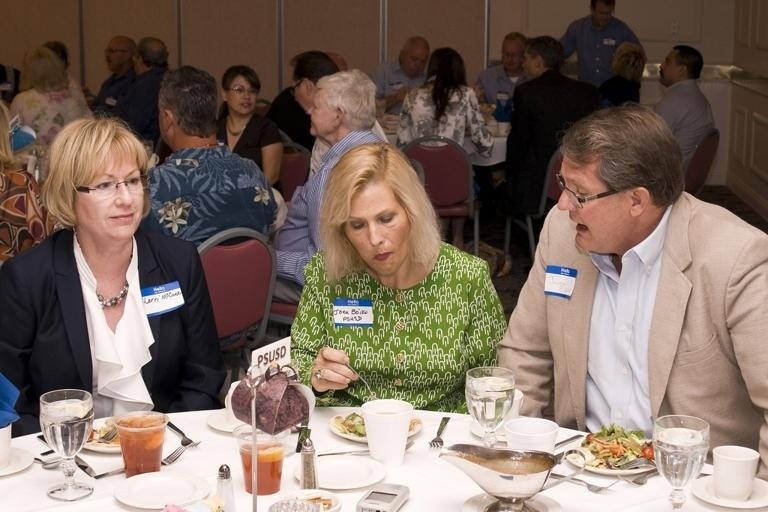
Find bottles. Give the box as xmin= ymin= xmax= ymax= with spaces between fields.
xmin=465 ymin=89 xmax=515 ymax=124
xmin=217 ymin=460 xmax=235 ymax=510
xmin=299 ymin=437 xmax=318 ymax=490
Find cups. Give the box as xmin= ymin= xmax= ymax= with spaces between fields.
xmin=113 ymin=409 xmax=168 ymax=479
xmin=503 ymin=417 xmax=558 ymax=455
xmin=236 ymin=423 xmax=286 ymax=494
xmin=0 ymin=418 xmax=15 ymax=462
xmin=360 ymin=400 xmax=415 ymax=462
xmin=470 ymin=389 xmax=523 ymax=435
xmin=713 ymin=446 xmax=761 ymax=500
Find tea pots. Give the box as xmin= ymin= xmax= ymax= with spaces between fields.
xmin=440 ymin=443 xmax=584 ymax=512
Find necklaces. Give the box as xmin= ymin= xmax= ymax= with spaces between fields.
xmin=75 ymin=235 xmax=133 ymax=308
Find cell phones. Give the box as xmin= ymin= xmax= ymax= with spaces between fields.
xmin=357 ymin=484 xmax=408 ymax=512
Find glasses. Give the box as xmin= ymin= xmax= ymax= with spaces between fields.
xmin=228 ymin=87 xmax=258 ymax=96
xmin=289 ymin=79 xmax=313 ymax=97
xmin=74 ymin=173 xmax=148 ymax=197
xmin=555 ymin=174 xmax=659 ymax=210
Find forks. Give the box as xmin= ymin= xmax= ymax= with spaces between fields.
xmin=429 ymin=414 xmax=450 ymax=448
xmin=551 ymin=472 xmax=622 ymax=495
xmin=169 ymin=421 xmax=202 ymax=450
xmin=622 ymin=468 xmax=658 ymax=488
xmin=160 ymin=446 xmax=186 ymax=466
xmin=86 ymin=428 xmax=118 ymax=445
xmin=38 ymin=436 xmax=97 ymax=478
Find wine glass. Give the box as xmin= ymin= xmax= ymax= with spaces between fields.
xmin=650 ymin=415 xmax=710 ymax=512
xmin=467 ymin=364 xmax=514 ymax=449
xmin=39 ymin=388 xmax=96 ymax=500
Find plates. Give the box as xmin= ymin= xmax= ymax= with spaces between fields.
xmin=284 ymin=455 xmax=390 ymax=490
xmin=113 ymin=469 xmax=213 ymax=511
xmin=264 ymin=490 xmax=343 ymax=512
xmin=206 ymin=405 xmax=252 ymax=434
xmin=83 ymin=415 xmax=122 ymax=453
xmin=0 ymin=448 xmax=34 ymax=479
xmin=332 ymin=411 xmax=423 ymax=444
xmin=696 ymin=475 xmax=767 ymax=511
xmin=565 ymin=430 xmax=660 ymax=473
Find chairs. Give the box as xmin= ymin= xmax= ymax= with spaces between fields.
xmin=4 ymin=89 xmax=724 ymax=402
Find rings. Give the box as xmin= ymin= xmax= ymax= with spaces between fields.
xmin=313 ymin=367 xmax=325 ymax=379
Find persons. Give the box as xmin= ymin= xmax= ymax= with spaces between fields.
xmin=1 ymin=111 xmax=229 ymax=438
xmin=289 ymin=140 xmax=508 ymax=415
xmin=497 ymin=102 xmax=768 ymax=483
